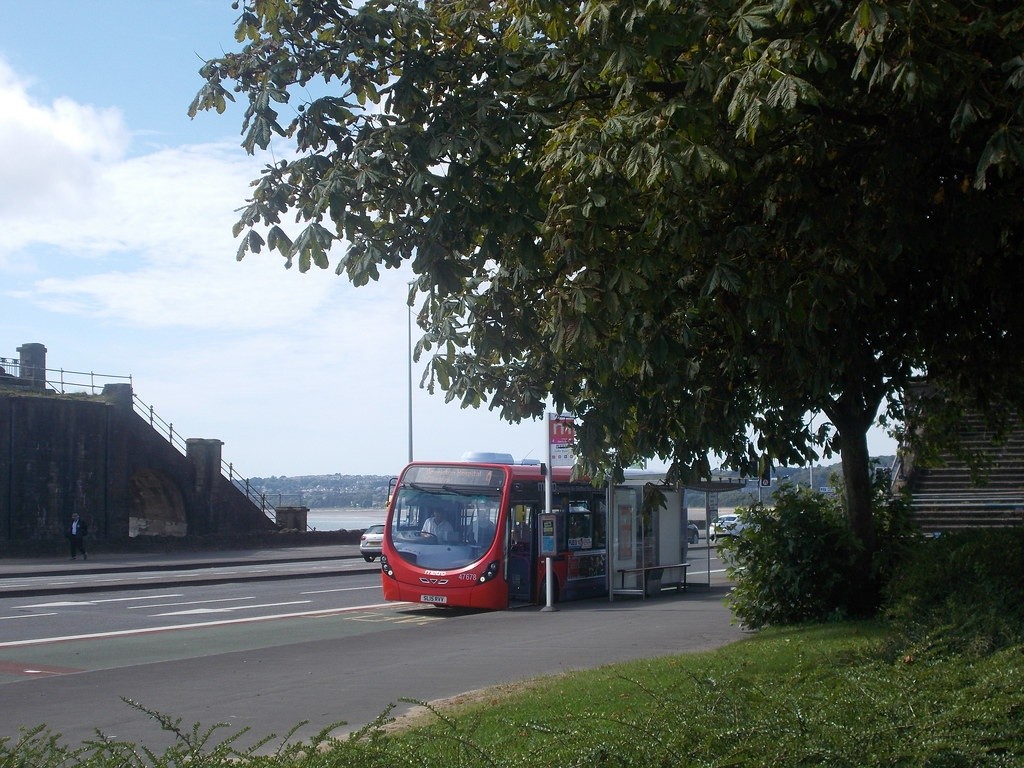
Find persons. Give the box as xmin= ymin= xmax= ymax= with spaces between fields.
xmin=421 ymin=508 xmax=453 ymax=539
xmin=469 ymin=510 xmax=494 ymax=545
xmin=69 ymin=512 xmax=88 ymax=561
xmin=510 ymin=522 xmax=530 ymax=545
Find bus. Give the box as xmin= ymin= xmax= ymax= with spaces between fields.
xmin=380 ymin=451 xmax=688 ymax=611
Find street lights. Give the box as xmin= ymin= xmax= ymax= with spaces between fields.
xmin=409 ymin=278 xmax=417 ymax=462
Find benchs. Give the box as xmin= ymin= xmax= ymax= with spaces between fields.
xmin=618 ymin=563 xmax=691 ymax=599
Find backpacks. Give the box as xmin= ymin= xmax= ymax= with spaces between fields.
xmin=81 ymin=520 xmax=88 ymax=536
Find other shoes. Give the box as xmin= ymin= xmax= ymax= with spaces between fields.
xmin=84 ymin=552 xmax=87 ymax=560
xmin=70 ymin=557 xmax=77 ymax=561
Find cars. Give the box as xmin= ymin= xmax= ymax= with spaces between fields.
xmin=686 ymin=521 xmax=699 ymax=544
xmin=360 ymin=522 xmax=386 ymax=562
xmin=710 ymin=515 xmax=747 ymax=541
